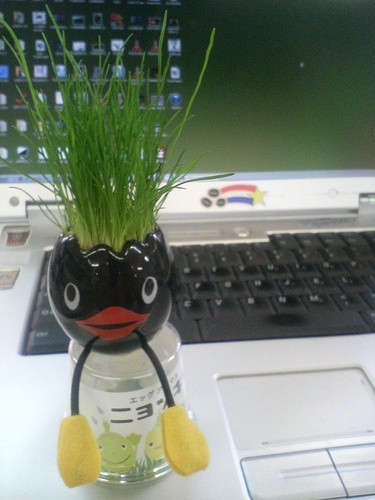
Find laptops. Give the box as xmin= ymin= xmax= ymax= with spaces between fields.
xmin=1 ymin=0 xmax=375 ymax=499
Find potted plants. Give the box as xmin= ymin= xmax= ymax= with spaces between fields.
xmin=0 ymin=11 xmax=215 ymax=490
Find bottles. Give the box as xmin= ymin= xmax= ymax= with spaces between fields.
xmin=70 ymin=321 xmax=185 ymax=485
